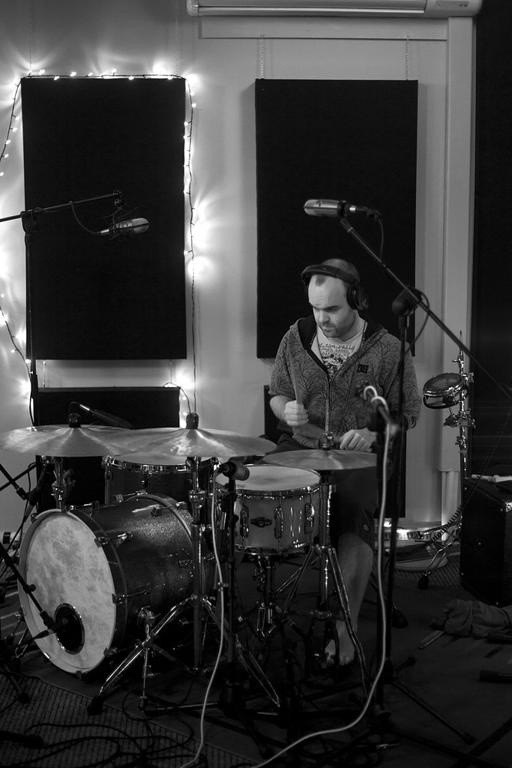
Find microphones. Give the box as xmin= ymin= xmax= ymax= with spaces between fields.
xmin=303 ymin=198 xmax=382 ymax=221
xmin=390 ymin=286 xmax=421 ymax=317
xmin=97 ymin=217 xmax=150 ymax=236
xmin=75 ymin=401 xmax=133 ymax=430
xmin=219 ymin=462 xmax=250 ymax=480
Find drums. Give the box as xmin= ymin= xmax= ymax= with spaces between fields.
xmin=209 ymin=465 xmax=326 ymax=557
xmin=18 ymin=494 xmax=215 ymax=678
xmin=106 ymin=458 xmax=220 ymax=532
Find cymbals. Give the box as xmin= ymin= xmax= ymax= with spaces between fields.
xmin=264 ymin=449 xmax=388 ymax=473
xmin=119 ymin=429 xmax=273 ymax=460
xmin=4 ymin=424 xmax=141 ymax=457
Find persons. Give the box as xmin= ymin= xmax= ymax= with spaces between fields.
xmin=268 ymin=257 xmax=422 ymax=667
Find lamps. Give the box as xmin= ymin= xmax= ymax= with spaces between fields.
xmin=186 ymin=0 xmax=483 ymax=18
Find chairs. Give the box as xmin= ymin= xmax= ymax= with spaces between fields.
xmin=264 ymin=385 xmax=407 ymax=620
xmin=32 ymin=386 xmax=182 ymax=512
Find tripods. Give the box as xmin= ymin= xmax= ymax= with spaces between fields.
xmin=297 ymin=222 xmax=512 ymax=746
xmin=86 ymin=483 xmax=368 ymax=766
xmin=412 ymin=428 xmax=468 ymax=591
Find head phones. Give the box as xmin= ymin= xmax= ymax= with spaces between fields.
xmin=302 ymin=265 xmax=371 ymax=311
